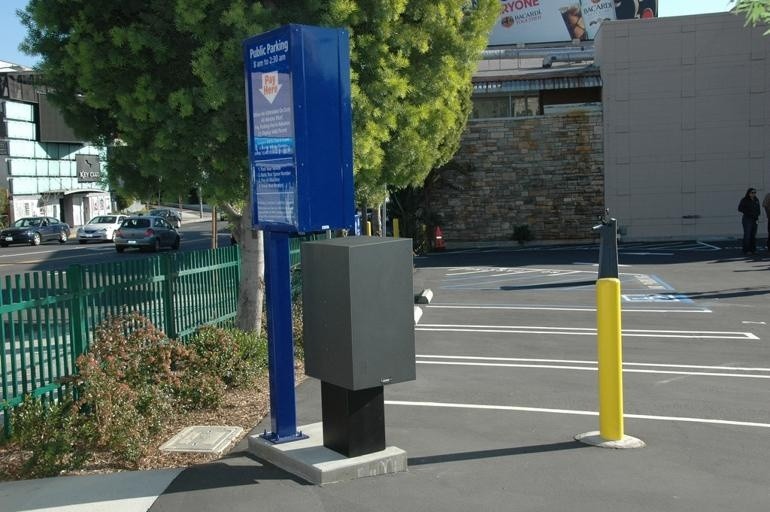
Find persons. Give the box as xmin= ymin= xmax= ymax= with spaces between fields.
xmin=762 ymin=192 xmax=770 ymax=249
xmin=737 ymin=188 xmax=761 ymax=255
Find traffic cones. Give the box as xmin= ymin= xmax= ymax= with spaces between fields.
xmin=433 ymin=225 xmax=447 ymax=251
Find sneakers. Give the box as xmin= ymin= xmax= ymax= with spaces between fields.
xmin=745 ymin=250 xmax=759 ymax=255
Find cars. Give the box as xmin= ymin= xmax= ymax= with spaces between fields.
xmin=76 ymin=214 xmax=131 ymax=245
xmin=146 ymin=208 xmax=182 ymax=229
xmin=0 ymin=216 xmax=72 ymax=249
xmin=114 ymin=215 xmax=182 ymax=253
